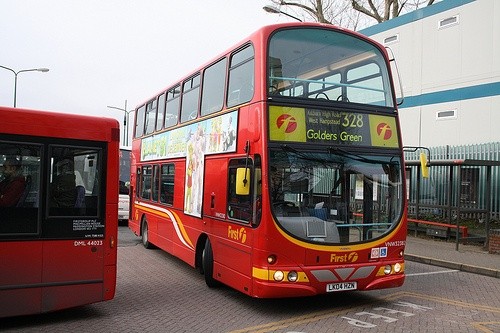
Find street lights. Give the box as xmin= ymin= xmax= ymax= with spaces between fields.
xmin=263 ymin=4 xmax=306 ymax=22
xmin=0 ymin=66 xmax=49 ymax=106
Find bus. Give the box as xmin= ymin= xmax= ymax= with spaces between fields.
xmin=130 ymin=22 xmax=431 ymax=300
xmin=1 ymin=107 xmax=119 ymax=318
xmin=119 ymin=148 xmax=132 ymax=221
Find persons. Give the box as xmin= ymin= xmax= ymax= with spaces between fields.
xmin=0 ymin=158 xmax=25 ymax=206
xmin=48 ymin=162 xmax=76 ymax=207
